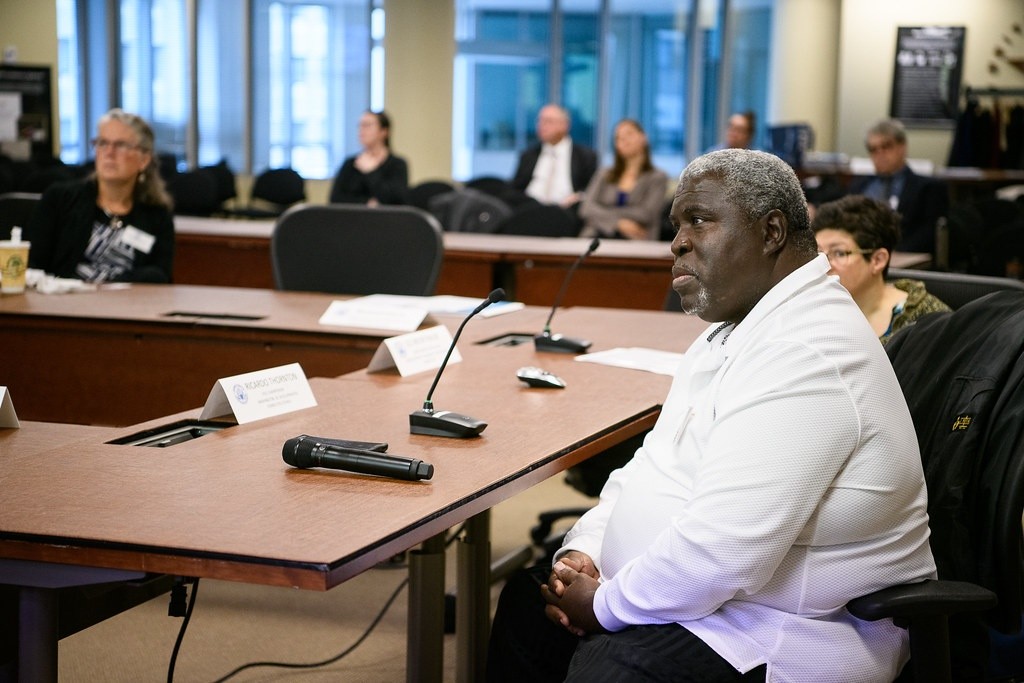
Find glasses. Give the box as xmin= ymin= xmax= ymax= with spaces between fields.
xmin=869 ymin=141 xmax=898 ymax=153
xmin=94 ymin=138 xmax=148 ymax=153
xmin=828 ymin=248 xmax=873 ymax=269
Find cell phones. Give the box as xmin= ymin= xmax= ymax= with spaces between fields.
xmin=302 ymin=435 xmax=388 ymax=453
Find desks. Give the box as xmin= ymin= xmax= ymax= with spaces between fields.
xmin=0 ymin=277 xmax=714 ymax=682
xmin=173 ymin=213 xmax=677 ymax=312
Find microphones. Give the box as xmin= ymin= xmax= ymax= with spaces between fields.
xmin=532 ymin=237 xmax=602 ymax=355
xmin=408 ymin=288 xmax=506 ymax=440
xmin=282 ymin=435 xmax=434 ymax=482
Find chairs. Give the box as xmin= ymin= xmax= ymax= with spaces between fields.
xmin=180 ymin=164 xmax=237 ymax=217
xmin=246 ymin=169 xmax=305 ymax=218
xmin=531 ymin=290 xmax=1024 ymax=683
xmin=269 ymin=204 xmax=445 ymax=296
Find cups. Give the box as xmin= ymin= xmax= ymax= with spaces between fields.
xmin=0 ymin=241 xmax=31 ymax=294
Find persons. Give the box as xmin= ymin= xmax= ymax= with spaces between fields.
xmin=703 ymin=110 xmax=755 ymax=149
xmin=858 ymin=119 xmax=934 ymax=253
xmin=810 ymin=195 xmax=954 ymax=343
xmin=327 ymin=110 xmax=408 ymax=206
xmin=483 ymin=149 xmax=939 ymax=683
xmin=25 ymin=109 xmax=176 ymax=284
xmin=509 ymin=104 xmax=596 ymax=205
xmin=578 ymin=120 xmax=666 ymax=239
